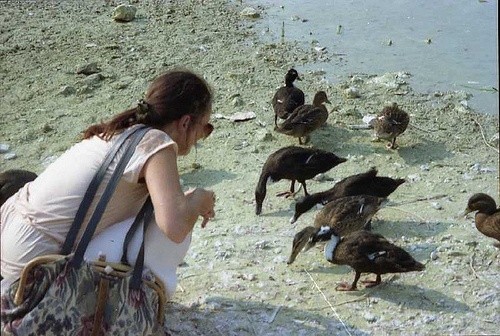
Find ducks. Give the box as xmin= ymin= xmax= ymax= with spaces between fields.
xmin=0 ymin=170 xmax=38 ymax=207
xmin=304 ymin=224 xmax=425 ymax=291
xmin=287 ymin=195 xmax=389 ymax=265
xmin=289 ymin=169 xmax=407 ymax=232
xmin=271 ymin=68 xmax=305 ymax=131
xmin=254 ymin=146 xmax=348 ymax=215
xmin=275 ymin=91 xmax=332 ymax=145
xmin=373 ymin=102 xmax=410 ymax=150
xmin=460 ymin=193 xmax=500 ymax=248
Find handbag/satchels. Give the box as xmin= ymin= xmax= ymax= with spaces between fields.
xmin=0 ymin=252 xmax=168 ymax=336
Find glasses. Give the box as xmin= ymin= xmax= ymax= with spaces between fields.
xmin=190 ymin=118 xmax=214 ymax=138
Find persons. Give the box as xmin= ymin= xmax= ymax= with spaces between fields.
xmin=0 ymin=67 xmax=220 ymax=336
